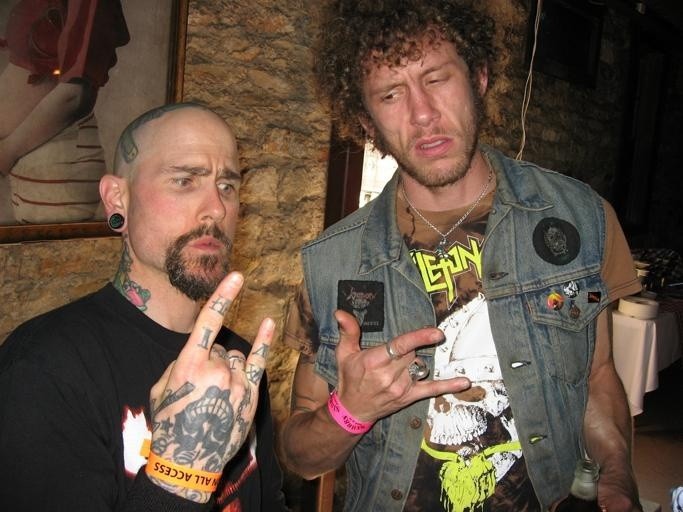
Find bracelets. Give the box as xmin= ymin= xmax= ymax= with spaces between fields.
xmin=327 ymin=390 xmax=370 ymax=435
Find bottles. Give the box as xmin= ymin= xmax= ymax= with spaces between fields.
xmin=555 ymin=459 xmax=603 ymax=511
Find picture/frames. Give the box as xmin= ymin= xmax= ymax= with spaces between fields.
xmin=0 ymin=0 xmax=191 ymax=248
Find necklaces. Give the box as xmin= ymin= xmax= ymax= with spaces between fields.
xmin=402 ymin=151 xmax=492 ymax=247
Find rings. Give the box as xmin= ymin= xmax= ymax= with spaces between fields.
xmin=385 ymin=342 xmax=402 ymax=361
xmin=407 ymin=362 xmax=429 ymax=383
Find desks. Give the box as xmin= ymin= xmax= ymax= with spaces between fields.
xmin=608 ymin=304 xmax=682 ymax=457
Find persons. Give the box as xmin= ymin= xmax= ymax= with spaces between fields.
xmin=0 ymin=0 xmax=130 ymax=225
xmin=279 ymin=0 xmax=643 ymax=512
xmin=0 ymin=102 xmax=292 ymax=512
